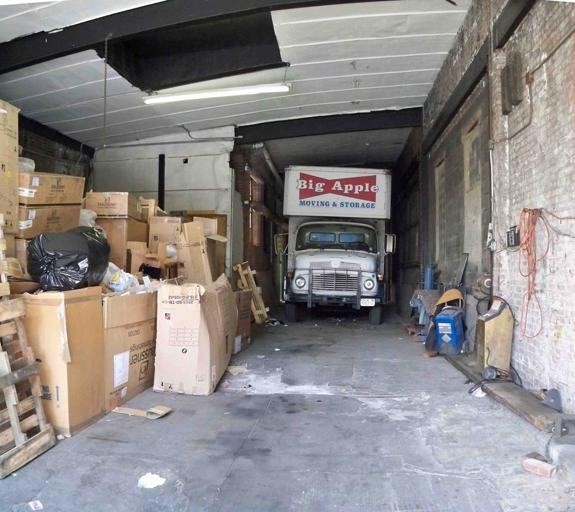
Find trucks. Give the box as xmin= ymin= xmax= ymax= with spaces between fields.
xmin=274 ymin=162 xmax=398 ymax=329
